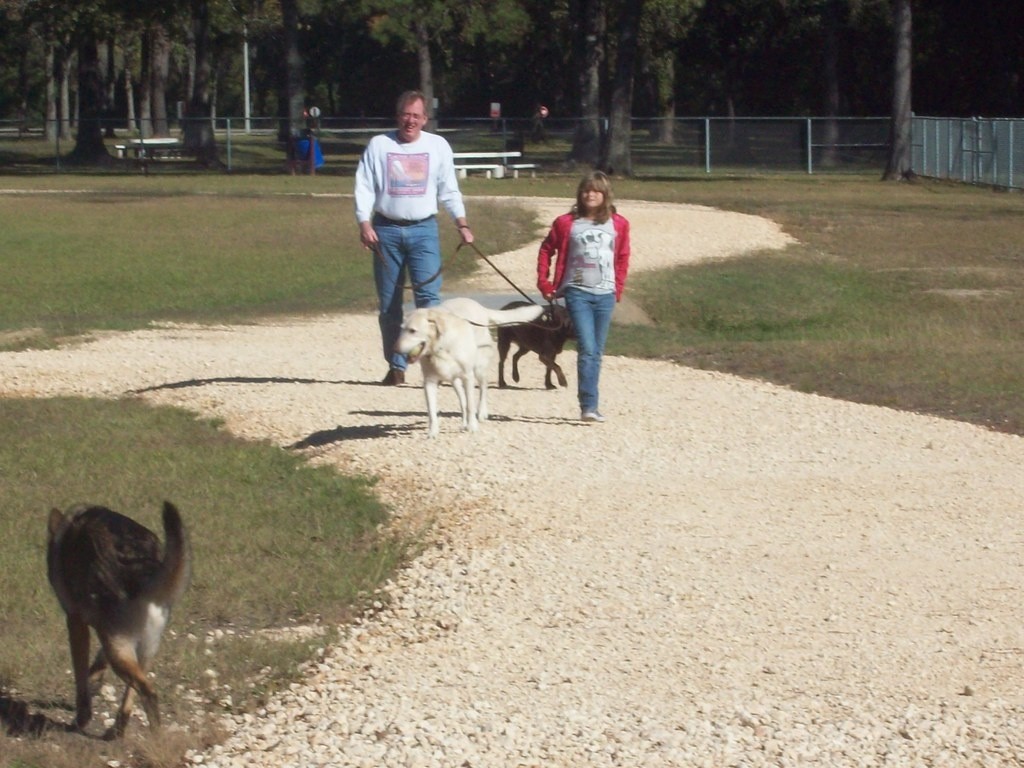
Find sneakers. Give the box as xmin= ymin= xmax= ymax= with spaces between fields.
xmin=582 ymin=410 xmax=606 ymax=422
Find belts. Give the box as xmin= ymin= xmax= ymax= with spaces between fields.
xmin=385 ymin=215 xmax=434 ymax=226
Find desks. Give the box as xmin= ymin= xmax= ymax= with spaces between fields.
xmin=453 ymin=152 xmax=522 ymax=178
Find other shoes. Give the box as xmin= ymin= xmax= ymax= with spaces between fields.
xmin=382 ymin=369 xmax=404 ymax=386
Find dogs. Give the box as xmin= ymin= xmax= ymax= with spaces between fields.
xmin=41 ymin=497 xmax=187 ymax=739
xmin=393 ymin=297 xmax=544 ymax=440
xmin=496 ymin=300 xmax=580 ymax=391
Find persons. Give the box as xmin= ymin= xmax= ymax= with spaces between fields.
xmin=354 ymin=91 xmax=472 ymax=383
xmin=536 ymin=170 xmax=630 ymax=421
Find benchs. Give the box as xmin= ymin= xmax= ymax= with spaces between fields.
xmin=115 ymin=137 xmax=227 ymax=176
xmin=454 ymin=163 xmax=537 ymax=179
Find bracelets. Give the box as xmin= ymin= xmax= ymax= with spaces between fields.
xmin=458 ymin=226 xmax=471 ymax=233
